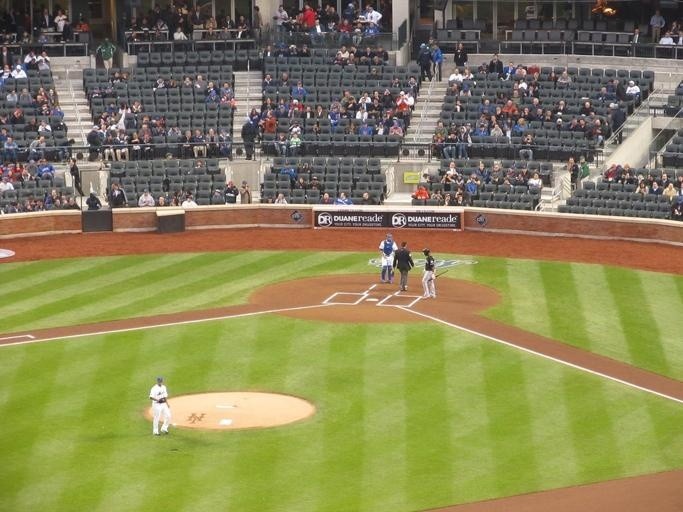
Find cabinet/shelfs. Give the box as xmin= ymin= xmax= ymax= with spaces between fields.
xmin=80 ymin=211 xmax=113 ymax=232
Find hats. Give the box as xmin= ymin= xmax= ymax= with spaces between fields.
xmin=16 ymin=65 xmax=21 ymax=71
xmin=422 ymin=248 xmax=431 ymax=252
xmin=157 ymin=377 xmax=164 ymax=384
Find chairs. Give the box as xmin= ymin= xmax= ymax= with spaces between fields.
xmin=0 ymin=18 xmax=682 ymax=219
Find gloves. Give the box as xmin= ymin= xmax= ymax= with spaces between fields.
xmin=431 ymin=274 xmax=435 ymax=280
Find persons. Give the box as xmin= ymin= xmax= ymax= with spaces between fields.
xmin=411 ymin=0 xmax=682 ymax=221
xmin=1 ymin=4 xmax=89 ymax=215
xmin=70 ymin=4 xmax=266 ymax=210
xmin=241 ymin=4 xmax=419 ymax=205
xmin=379 ymin=233 xmax=436 ymax=299
xmin=149 ymin=377 xmax=170 ymax=436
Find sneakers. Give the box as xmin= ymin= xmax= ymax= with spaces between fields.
xmin=153 ymin=429 xmax=169 ymax=436
xmin=381 ymin=279 xmax=393 ymax=284
xmin=399 ymin=286 xmax=408 ymax=291
xmin=422 ymin=292 xmax=435 ymax=299
